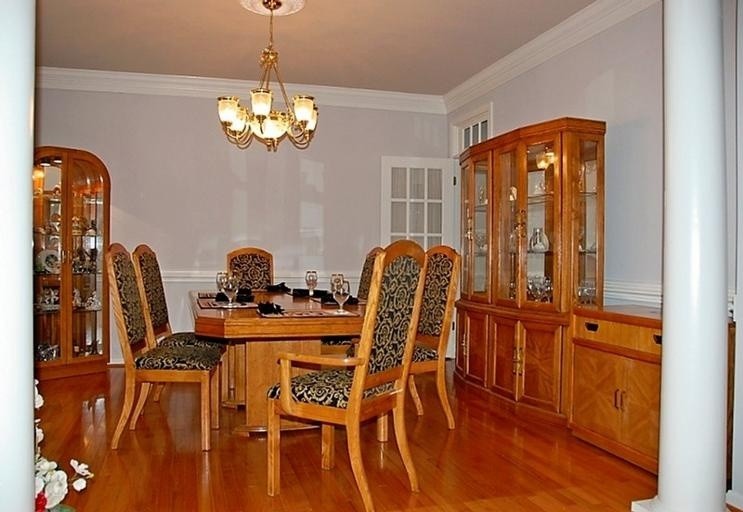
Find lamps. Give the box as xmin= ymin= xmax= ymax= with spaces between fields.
xmin=534 ymin=142 xmax=557 ymax=171
xmin=218 ymin=0 xmax=319 ymax=152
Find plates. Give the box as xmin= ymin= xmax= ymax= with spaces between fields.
xmin=38 ymin=249 xmax=60 ymax=274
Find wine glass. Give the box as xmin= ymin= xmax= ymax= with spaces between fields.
xmin=214 ymin=272 xmax=239 ymax=309
xmin=328 ymin=273 xmax=351 ymax=315
xmin=304 ymin=270 xmax=318 ymax=296
xmin=474 ymin=233 xmax=486 ymax=255
xmin=505 ymin=273 xmax=595 ymax=305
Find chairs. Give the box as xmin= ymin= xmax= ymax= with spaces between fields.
xmin=357 ymin=247 xmax=384 ymax=305
xmin=268 ymin=239 xmax=429 ymax=511
xmin=404 ymin=241 xmax=460 ymax=429
xmin=105 ymin=244 xmax=226 ymax=451
xmin=224 ymin=247 xmax=275 ymax=290
xmin=132 ymin=244 xmax=234 ymax=410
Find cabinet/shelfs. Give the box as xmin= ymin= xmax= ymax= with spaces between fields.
xmin=501 ymin=117 xmax=603 ymax=319
xmin=571 ymin=305 xmax=736 ymax=487
xmin=460 ymin=140 xmax=499 ymax=313
xmin=454 ymin=305 xmax=489 ymax=393
xmin=33 ymin=148 xmax=111 ymax=379
xmin=489 ymin=312 xmax=574 ymax=430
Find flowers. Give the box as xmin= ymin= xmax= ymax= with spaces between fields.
xmin=36 ymin=377 xmax=95 ymax=511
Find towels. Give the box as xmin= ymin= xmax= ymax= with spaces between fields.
xmin=215 ymin=283 xmax=359 ymax=316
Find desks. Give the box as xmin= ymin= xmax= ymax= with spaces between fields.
xmin=179 ymin=284 xmax=396 ymax=434
